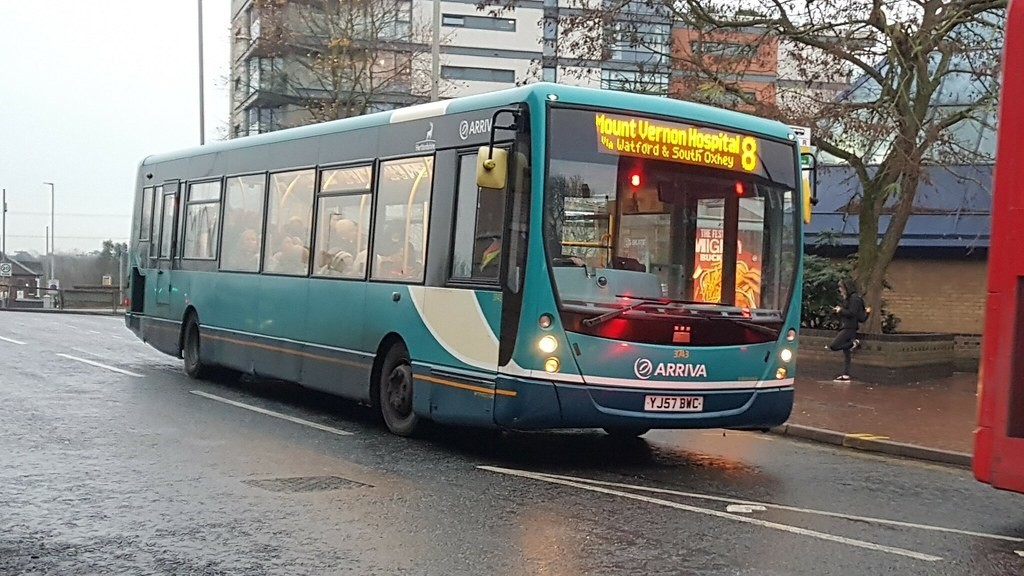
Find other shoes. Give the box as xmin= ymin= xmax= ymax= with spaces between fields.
xmin=850 ymin=339 xmax=859 ymax=351
xmin=834 ymin=375 xmax=851 ymax=382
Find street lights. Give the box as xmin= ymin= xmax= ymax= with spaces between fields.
xmin=45 ymin=182 xmax=55 ymax=310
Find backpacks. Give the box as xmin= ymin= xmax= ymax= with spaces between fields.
xmin=855 ymin=295 xmax=870 ymax=323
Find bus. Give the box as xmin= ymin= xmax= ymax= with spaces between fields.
xmin=121 ymin=82 xmax=819 ymax=437
xmin=972 ymin=0 xmax=1024 ymax=493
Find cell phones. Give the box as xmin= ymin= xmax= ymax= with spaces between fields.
xmin=829 ymin=305 xmax=834 ymax=309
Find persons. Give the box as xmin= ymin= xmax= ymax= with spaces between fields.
xmin=828 ymin=275 xmax=871 ymax=383
xmin=238 ymin=213 xmax=416 ymax=279
xmin=474 ymin=210 xmax=585 ymax=276
